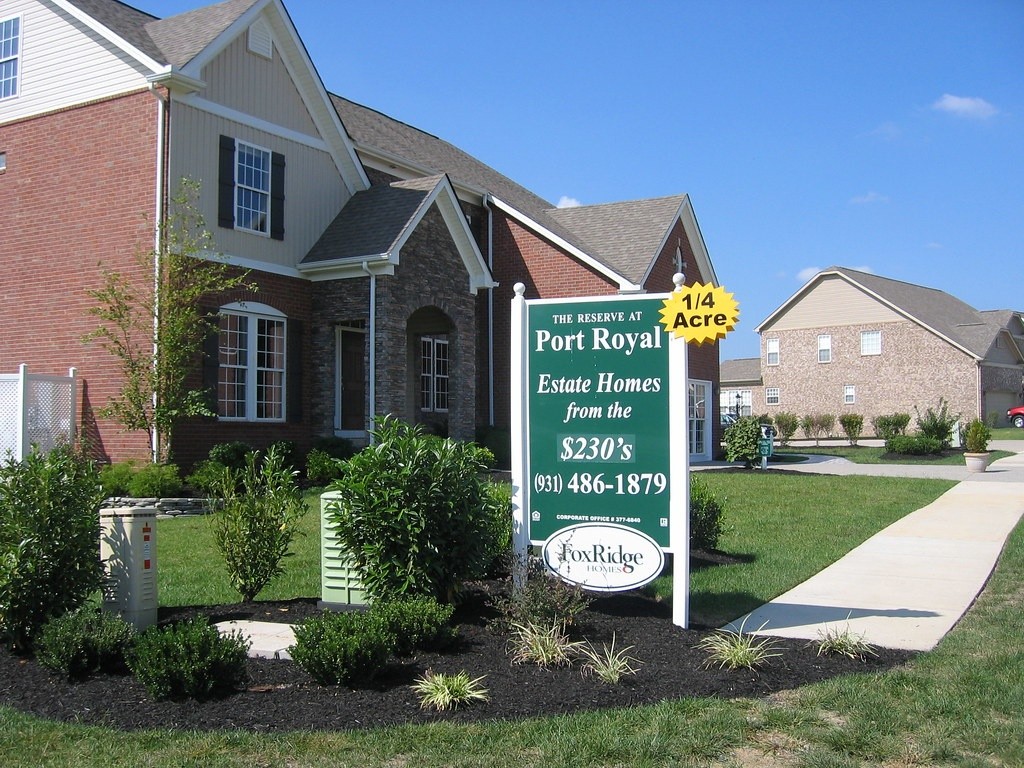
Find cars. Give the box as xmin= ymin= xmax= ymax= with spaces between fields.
xmin=719 ymin=413 xmax=778 ymax=438
xmin=1004 ymin=407 xmax=1024 ymax=428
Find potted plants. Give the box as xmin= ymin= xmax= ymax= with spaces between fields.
xmin=963 ymin=418 xmax=993 ymax=473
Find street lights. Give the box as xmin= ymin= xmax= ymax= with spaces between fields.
xmin=735 ymin=393 xmax=741 ymax=421
